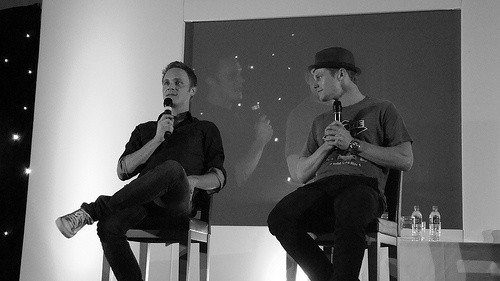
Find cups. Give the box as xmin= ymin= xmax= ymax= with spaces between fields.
xmin=400 ymin=216 xmax=426 ymax=240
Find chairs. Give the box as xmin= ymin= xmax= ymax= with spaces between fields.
xmin=285 ymin=168 xmax=404 ymax=281
xmin=100 ymin=194 xmax=214 ymax=281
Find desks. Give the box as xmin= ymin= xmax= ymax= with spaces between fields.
xmin=394 ymin=238 xmax=500 ymax=281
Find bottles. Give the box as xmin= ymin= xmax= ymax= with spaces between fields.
xmin=411 ymin=205 xmax=422 ymax=240
xmin=429 ymin=205 xmax=441 ymax=241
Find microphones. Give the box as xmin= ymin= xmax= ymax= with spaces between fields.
xmin=249 ymin=100 xmax=262 ymax=119
xmin=163 ymin=98 xmax=173 ymax=136
xmin=332 ymin=100 xmax=342 ymax=150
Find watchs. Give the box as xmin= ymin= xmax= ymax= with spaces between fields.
xmin=348 ymin=139 xmax=360 ymax=153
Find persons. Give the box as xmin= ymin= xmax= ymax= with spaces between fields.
xmin=194 ymin=53 xmax=336 ymax=224
xmin=267 ymin=47 xmax=412 ymax=281
xmin=55 ymin=62 xmax=227 ymax=281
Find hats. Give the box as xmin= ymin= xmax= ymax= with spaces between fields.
xmin=308 ymin=47 xmax=361 ymax=77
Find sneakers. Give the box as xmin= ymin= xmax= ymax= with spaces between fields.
xmin=56 ymin=207 xmax=94 ymax=238
